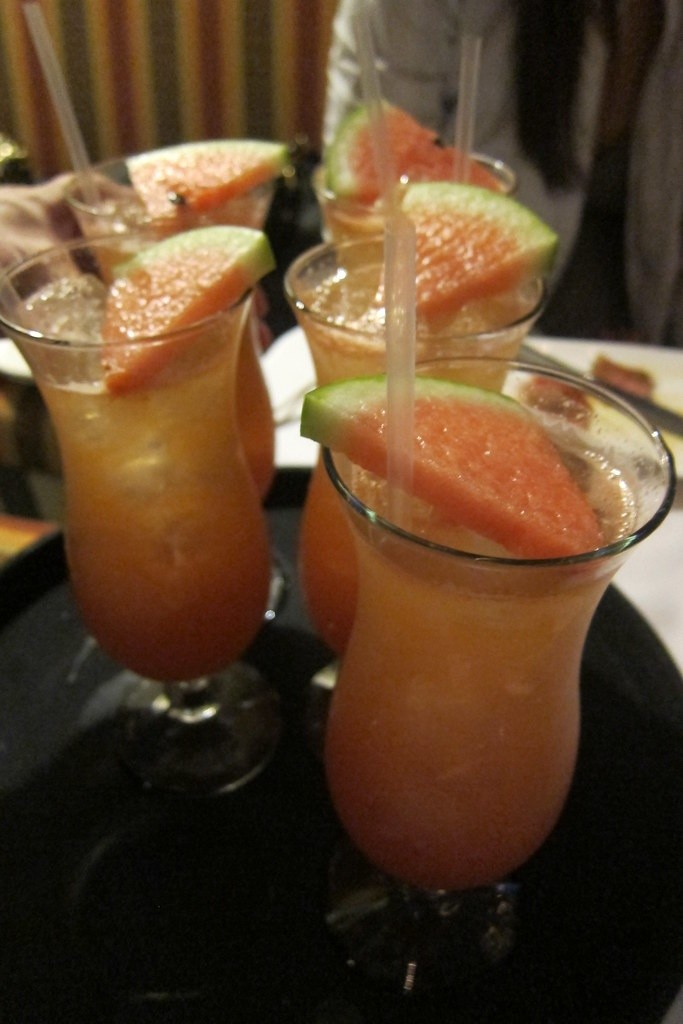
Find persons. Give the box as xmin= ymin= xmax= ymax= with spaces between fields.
xmin=323 ymin=0 xmax=683 ymax=348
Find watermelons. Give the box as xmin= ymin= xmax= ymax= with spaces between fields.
xmin=120 ymin=136 xmax=290 ymax=227
xmin=329 ymin=97 xmax=505 ymax=203
xmin=378 ymin=180 xmax=557 ymax=327
xmin=104 ymin=226 xmax=275 ymax=394
xmin=296 ymin=374 xmax=604 ymax=575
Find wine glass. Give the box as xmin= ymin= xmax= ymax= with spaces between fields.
xmin=65 ymin=156 xmax=288 ymax=626
xmin=312 ymin=150 xmax=516 ymax=240
xmin=1 ymin=234 xmax=283 ymax=799
xmin=285 ymin=240 xmax=550 ymax=688
xmin=323 ymin=357 xmax=675 ymax=997
